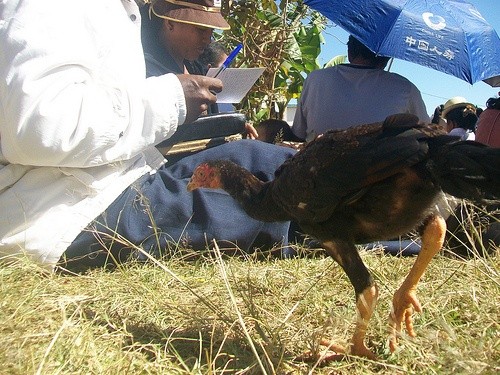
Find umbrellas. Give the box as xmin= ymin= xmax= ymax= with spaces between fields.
xmin=296 ymin=0 xmax=500 ymax=85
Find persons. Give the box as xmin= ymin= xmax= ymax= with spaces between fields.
xmin=289 ymin=33 xmax=435 ymax=143
xmin=430 ymin=91 xmax=500 ymax=148
xmin=138 ymin=0 xmax=295 ymax=144
xmin=0 ymin=0 xmax=500 ymax=277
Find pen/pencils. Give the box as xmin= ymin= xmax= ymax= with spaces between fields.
xmin=208 ymin=42 xmax=244 ymax=77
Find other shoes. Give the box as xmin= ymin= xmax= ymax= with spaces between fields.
xmin=446 ymin=204 xmax=500 ymax=259
xmin=473 ymin=200 xmax=500 ymax=222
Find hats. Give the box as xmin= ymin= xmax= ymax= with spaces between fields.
xmin=442 ymin=97 xmax=473 ymax=119
xmin=152 ymin=0 xmax=231 ymax=30
xmin=257 ymin=118 xmax=305 ymax=142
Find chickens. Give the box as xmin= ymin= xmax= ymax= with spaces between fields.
xmin=186 ymin=112 xmax=500 ymax=365
